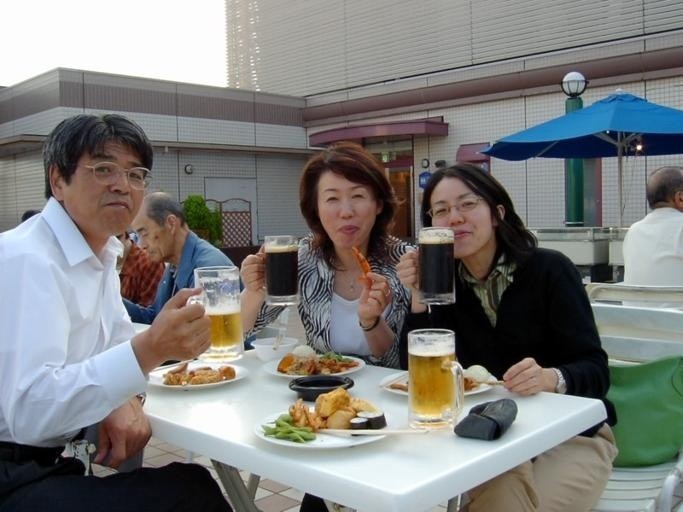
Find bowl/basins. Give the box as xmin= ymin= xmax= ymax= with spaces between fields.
xmin=251 ymin=338 xmax=295 ymax=364
xmin=286 ymin=375 xmax=352 ymax=401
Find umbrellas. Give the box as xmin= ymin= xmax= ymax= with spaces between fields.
xmin=480 ymin=90 xmax=683 ymax=279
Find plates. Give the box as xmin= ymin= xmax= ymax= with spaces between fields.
xmin=380 ymin=374 xmax=497 ymax=396
xmin=148 ymin=365 xmax=250 ymax=391
xmin=251 ymin=406 xmax=387 ymax=447
xmin=262 ymin=355 xmax=367 ymax=377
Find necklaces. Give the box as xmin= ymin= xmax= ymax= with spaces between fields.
xmin=337 ymin=268 xmax=367 ymax=295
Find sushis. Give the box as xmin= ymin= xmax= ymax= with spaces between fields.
xmin=350 ymin=411 xmax=386 ymax=429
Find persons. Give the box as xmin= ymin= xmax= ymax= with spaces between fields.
xmin=396 ymin=164 xmax=616 ymax=512
xmin=233 ymin=141 xmax=417 ymax=511
xmin=82 ymin=191 xmax=256 ymax=471
xmin=21 ymin=209 xmax=41 ymax=222
xmin=622 ymin=167 xmax=683 ymax=310
xmin=0 ymin=112 xmax=233 ymax=512
xmin=115 ymin=230 xmax=166 ymax=307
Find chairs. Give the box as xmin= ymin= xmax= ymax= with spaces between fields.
xmin=539 ymin=304 xmax=681 ymax=509
xmin=585 ymin=281 xmax=682 ymax=311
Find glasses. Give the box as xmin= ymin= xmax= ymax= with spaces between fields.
xmin=79 ymin=162 xmax=154 ymax=190
xmin=427 ymin=196 xmax=483 ymax=219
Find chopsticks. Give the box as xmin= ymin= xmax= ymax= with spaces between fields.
xmin=320 ymin=427 xmax=428 ymax=438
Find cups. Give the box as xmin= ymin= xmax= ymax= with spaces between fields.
xmin=185 ymin=264 xmax=243 ymax=363
xmin=260 ymin=233 xmax=302 ymax=310
xmin=407 ymin=328 xmax=464 ymax=429
xmin=411 ymin=227 xmax=456 ymax=306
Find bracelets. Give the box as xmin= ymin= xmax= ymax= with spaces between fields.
xmin=359 ymin=315 xmax=381 ymax=331
xmin=137 ymin=394 xmax=146 ymax=405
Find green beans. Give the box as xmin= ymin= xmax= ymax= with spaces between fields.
xmin=261 ymin=413 xmax=316 ymax=443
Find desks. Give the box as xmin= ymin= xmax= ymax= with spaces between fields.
xmin=130 ymin=341 xmax=609 ymax=511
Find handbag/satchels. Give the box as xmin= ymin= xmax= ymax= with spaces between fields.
xmin=605 ymin=357 xmax=682 ymax=467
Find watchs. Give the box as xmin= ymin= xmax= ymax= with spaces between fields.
xmin=548 ymin=364 xmax=568 ymax=392
